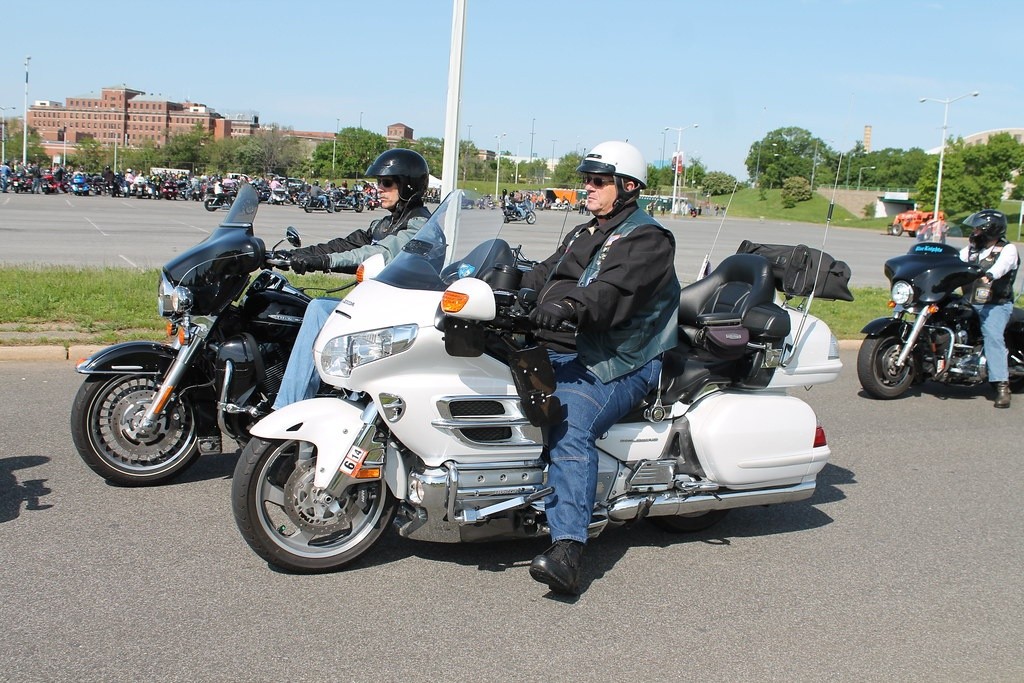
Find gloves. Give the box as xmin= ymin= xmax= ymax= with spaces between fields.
xmin=290 ymin=254 xmax=330 ymax=275
xmin=975 ymin=276 xmax=989 ymax=288
xmin=527 ymin=300 xmax=575 ymax=332
xmin=272 ymin=250 xmax=293 ymax=271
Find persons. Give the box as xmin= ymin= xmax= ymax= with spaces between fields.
xmin=959 ymin=210 xmax=1021 ymax=408
xmin=266 ymin=148 xmax=446 ymax=411
xmin=520 ymin=140 xmax=681 ymax=595
xmin=78 ymin=165 xmax=146 ymax=198
xmin=215 ymin=176 xmax=233 ymax=204
xmin=270 ymin=177 xmax=296 ymax=205
xmin=1 ymin=159 xmax=42 ymax=194
xmin=648 ymin=201 xmax=726 ymax=217
xmin=297 ymin=176 xmax=347 ymax=208
xmin=48 ymin=164 xmax=74 ymax=194
xmin=528 ymin=192 xmax=590 ymax=216
xmin=509 ymin=192 xmax=526 ymax=219
xmin=502 ymin=189 xmax=517 ymax=214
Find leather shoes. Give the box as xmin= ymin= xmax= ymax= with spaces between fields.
xmin=994 ymin=383 xmax=1011 ymax=408
xmin=530 ymin=540 xmax=583 ymax=596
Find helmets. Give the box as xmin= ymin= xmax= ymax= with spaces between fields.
xmin=510 ymin=192 xmax=514 ymax=197
xmin=969 ymin=209 xmax=1007 ymax=249
xmin=314 ymin=181 xmax=318 ymax=186
xmin=502 ymin=189 xmax=507 ymax=194
xmin=575 ymin=140 xmax=647 ymax=189
xmin=365 ymin=148 xmax=429 ymax=200
xmin=4 ymin=160 xmax=9 ymax=166
xmin=127 ymin=169 xmax=132 ymax=172
xmin=217 ymin=177 xmax=222 ymax=180
xmin=105 ymin=165 xmax=110 ymax=169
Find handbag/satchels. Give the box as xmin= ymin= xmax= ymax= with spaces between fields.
xmin=735 ymin=240 xmax=854 ymax=302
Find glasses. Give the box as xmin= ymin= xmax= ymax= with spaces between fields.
xmin=377 ymin=178 xmax=398 ymax=187
xmin=584 ymin=176 xmax=630 ymax=186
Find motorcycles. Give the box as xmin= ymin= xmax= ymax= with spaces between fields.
xmin=855 ymin=220 xmax=1024 ymax=400
xmin=227 ymin=148 xmax=844 ymax=576
xmin=68 ymin=177 xmax=544 ymax=490
xmin=0 ymin=159 xmax=382 ymax=214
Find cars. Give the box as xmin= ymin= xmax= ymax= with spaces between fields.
xmin=887 ymin=210 xmax=946 ymax=239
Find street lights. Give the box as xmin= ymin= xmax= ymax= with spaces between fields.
xmin=665 ymin=123 xmax=699 ymax=214
xmin=852 ymin=166 xmax=877 ymax=215
xmin=755 ymin=104 xmax=767 ymax=185
xmin=918 ymin=91 xmax=980 ymax=221
